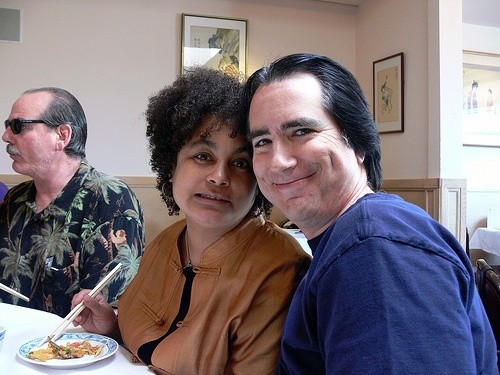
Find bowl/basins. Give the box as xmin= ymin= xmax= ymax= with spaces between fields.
xmin=0 ymin=326 xmax=6 ymax=342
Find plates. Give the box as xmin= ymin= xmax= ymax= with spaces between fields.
xmin=18 ymin=334 xmax=119 ymax=369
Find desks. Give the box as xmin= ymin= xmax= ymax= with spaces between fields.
xmin=469 ymin=227 xmax=500 ymax=264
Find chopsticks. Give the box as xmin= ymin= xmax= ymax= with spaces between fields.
xmin=38 ymin=263 xmax=122 ymax=348
xmin=0 ymin=283 xmax=29 ymax=302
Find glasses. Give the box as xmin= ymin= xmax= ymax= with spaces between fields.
xmin=4 ymin=119 xmax=51 ymax=135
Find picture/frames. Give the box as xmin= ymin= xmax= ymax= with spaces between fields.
xmin=180 ymin=13 xmax=248 ymax=82
xmin=372 ymin=52 xmax=404 ymax=133
xmin=462 ymin=50 xmax=500 ymax=147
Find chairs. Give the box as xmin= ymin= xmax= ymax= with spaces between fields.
xmin=476 ymin=259 xmax=500 ymax=349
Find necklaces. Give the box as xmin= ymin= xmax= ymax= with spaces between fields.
xmin=185 ymin=229 xmax=195 ymax=267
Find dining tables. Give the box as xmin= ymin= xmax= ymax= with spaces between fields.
xmin=0 ymin=302 xmax=157 ymax=375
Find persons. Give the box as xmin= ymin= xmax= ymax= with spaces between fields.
xmin=72 ymin=66 xmax=313 ymax=375
xmin=0 ymin=88 xmax=146 ymax=321
xmin=242 ymin=53 xmax=499 ymax=375
